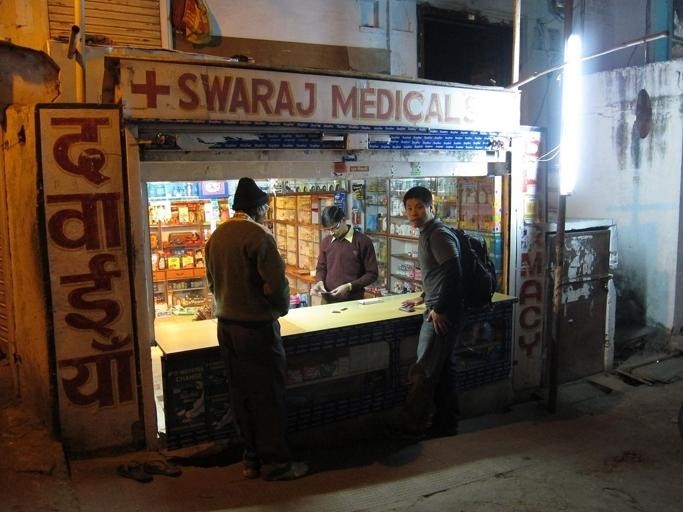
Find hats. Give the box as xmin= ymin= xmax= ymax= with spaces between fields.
xmin=231 ymin=177 xmax=269 ymax=211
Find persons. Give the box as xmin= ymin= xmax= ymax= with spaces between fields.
xmin=203 ymin=176 xmax=324 ymax=484
xmin=380 ymin=184 xmax=479 ymax=446
xmin=306 ymin=204 xmax=380 ymax=306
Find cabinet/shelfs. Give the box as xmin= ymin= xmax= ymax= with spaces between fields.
xmin=149 ymin=176 xmax=233 ymax=307
xmin=352 ymin=178 xmax=498 ymax=303
xmin=262 ymin=179 xmax=347 ymax=307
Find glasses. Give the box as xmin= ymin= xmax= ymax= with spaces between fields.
xmin=322 ymin=221 xmax=340 ymax=234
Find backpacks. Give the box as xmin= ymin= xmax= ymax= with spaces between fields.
xmin=422 ymin=222 xmax=496 ymax=313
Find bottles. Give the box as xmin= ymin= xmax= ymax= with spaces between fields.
xmin=460 ymin=187 xmax=493 ymax=204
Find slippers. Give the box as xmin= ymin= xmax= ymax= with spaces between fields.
xmin=116 ymin=456 xmax=182 ymax=482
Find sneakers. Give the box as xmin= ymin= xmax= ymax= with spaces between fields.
xmin=388 ymin=424 xmax=456 ymax=451
xmin=242 ymin=449 xmax=310 ymax=482
